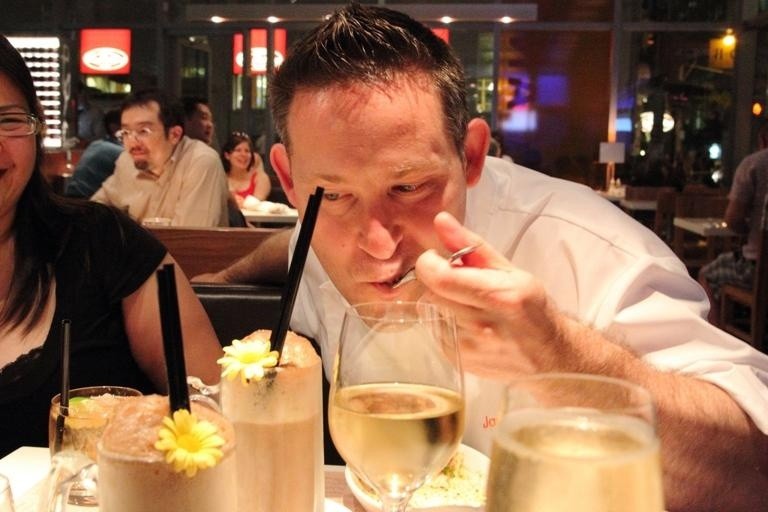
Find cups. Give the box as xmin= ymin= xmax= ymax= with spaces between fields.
xmin=485 ymin=371 xmax=666 ymax=512
xmin=46 ymin=383 xmax=146 ymax=508
xmin=215 ymin=356 xmax=325 ymax=512
xmin=95 ymin=421 xmax=241 ymax=512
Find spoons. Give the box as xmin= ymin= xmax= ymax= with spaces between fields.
xmin=389 ymin=238 xmax=485 ymax=290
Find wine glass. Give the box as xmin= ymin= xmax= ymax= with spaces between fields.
xmin=325 ymin=300 xmax=468 ymax=512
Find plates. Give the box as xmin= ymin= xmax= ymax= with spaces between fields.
xmin=340 ymin=441 xmax=495 ymax=511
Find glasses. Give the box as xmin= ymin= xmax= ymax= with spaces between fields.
xmin=0 ymin=111 xmax=38 ymax=136
xmin=229 ymin=130 xmax=249 ymax=139
xmin=114 ymin=128 xmax=166 ymax=142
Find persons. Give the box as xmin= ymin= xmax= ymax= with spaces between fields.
xmin=267 ymin=0 xmax=768 ymax=510
xmin=492 ymin=131 xmax=512 ymax=162
xmin=696 ymin=116 xmax=768 ymax=330
xmin=0 ymin=30 xmax=228 ymax=461
xmin=486 ymin=137 xmax=502 ymax=159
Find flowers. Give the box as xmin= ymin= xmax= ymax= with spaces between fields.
xmin=217 ymin=339 xmax=278 ymax=387
xmin=154 ymin=408 xmax=225 ymax=479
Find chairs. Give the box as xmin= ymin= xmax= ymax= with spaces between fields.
xmin=605 ymin=181 xmax=768 ymax=353
xmin=142 ymin=222 xmax=292 ymax=284
xmin=197 ymin=293 xmax=288 ymax=350
